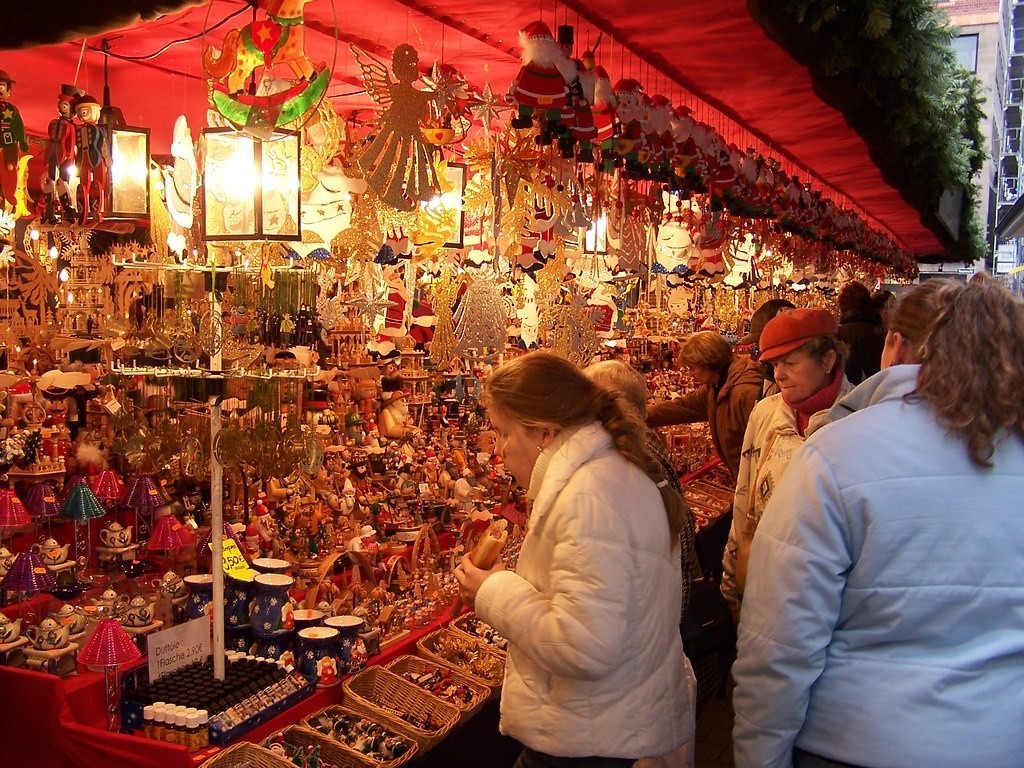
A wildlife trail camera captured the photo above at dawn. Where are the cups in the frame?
[182,560,367,686]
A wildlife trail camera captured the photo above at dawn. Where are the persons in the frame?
[1,69,112,227]
[455,353,701,768]
[9,344,105,480]
[146,351,514,559]
[573,271,1024,768]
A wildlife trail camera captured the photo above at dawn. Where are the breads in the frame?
[469,519,508,570]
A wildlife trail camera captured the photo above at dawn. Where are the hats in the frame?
[758,308,838,361]
[736,299,796,346]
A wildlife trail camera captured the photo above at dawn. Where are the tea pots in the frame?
[0,572,189,651]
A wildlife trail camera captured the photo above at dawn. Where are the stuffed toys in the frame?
[377,262,438,355]
[504,22,919,280]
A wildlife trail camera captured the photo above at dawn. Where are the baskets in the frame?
[416,628,507,700]
[679,462,737,536]
[383,654,492,722]
[198,741,300,768]
[448,611,509,657]
[299,704,419,768]
[258,724,380,768]
[341,665,461,757]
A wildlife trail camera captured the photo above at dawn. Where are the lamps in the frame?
[583,210,608,255]
[194,123,305,242]
[44,38,150,218]
[413,161,468,246]
